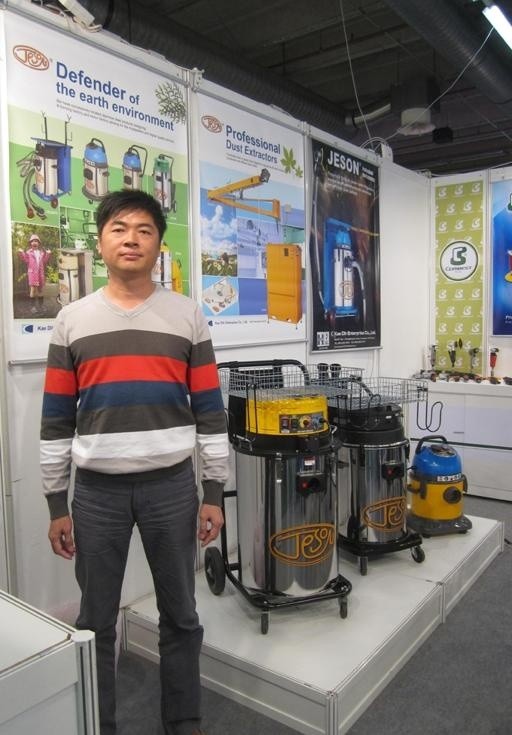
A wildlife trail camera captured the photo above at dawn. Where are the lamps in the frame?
[479,0,512,51]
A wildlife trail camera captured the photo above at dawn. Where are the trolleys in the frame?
[300,363,428,574]
[204,361,350,636]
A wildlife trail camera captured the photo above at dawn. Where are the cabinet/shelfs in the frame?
[404,379,512,501]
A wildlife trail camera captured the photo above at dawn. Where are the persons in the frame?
[38,188,231,735]
[17,235,51,315]
